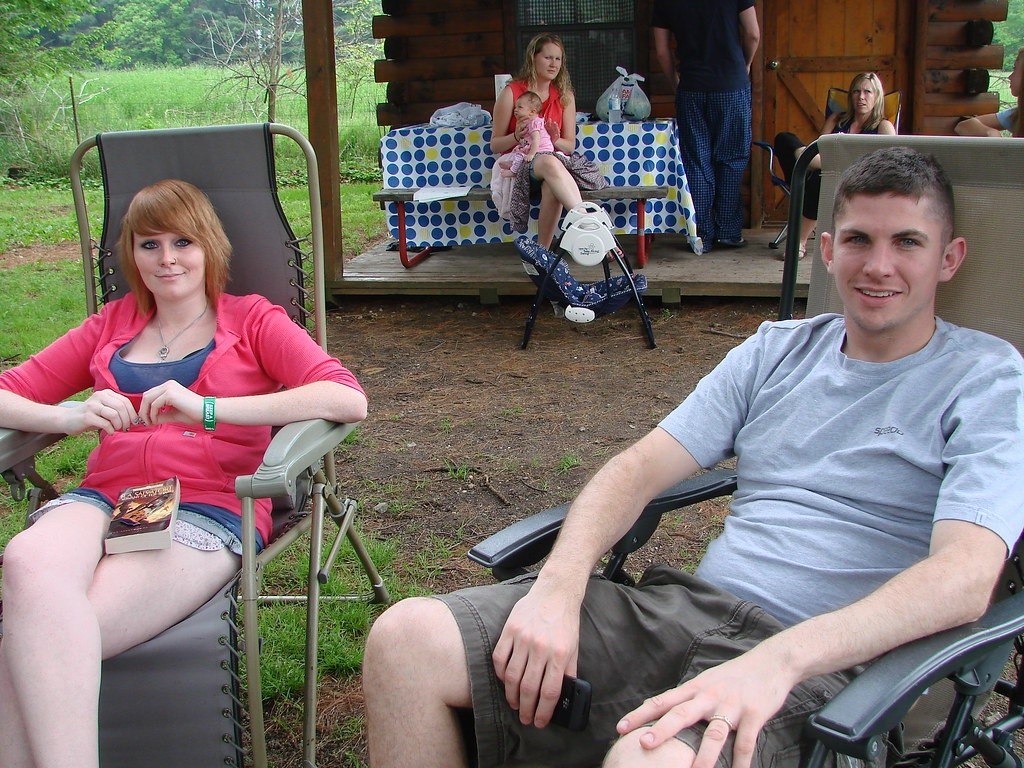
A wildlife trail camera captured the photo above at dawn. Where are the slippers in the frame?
[781,247,807,261]
[718,238,749,247]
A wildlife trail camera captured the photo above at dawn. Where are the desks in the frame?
[380,116,703,258]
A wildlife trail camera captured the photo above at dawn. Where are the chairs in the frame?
[514,201,655,348]
[752,87,903,248]
[468,133,1024,768]
[0,122,387,768]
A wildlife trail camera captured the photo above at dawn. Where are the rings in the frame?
[98,405,105,416]
[708,715,733,730]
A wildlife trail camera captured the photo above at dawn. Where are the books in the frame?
[104,476,181,554]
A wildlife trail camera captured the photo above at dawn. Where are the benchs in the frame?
[373,185,668,267]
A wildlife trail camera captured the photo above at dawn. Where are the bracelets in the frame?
[203,397,216,431]
[513,131,520,143]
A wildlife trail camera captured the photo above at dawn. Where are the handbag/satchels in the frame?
[596,67,651,121]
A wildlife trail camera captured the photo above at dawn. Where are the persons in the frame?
[499,91,554,178]
[774,71,896,260]
[362,147,1023,768]
[490,31,624,262]
[0,180,369,768]
[652,0,761,252]
[955,47,1024,140]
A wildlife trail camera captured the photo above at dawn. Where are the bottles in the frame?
[464,113,490,128]
[609,88,621,123]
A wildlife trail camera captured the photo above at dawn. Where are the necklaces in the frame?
[154,296,208,360]
